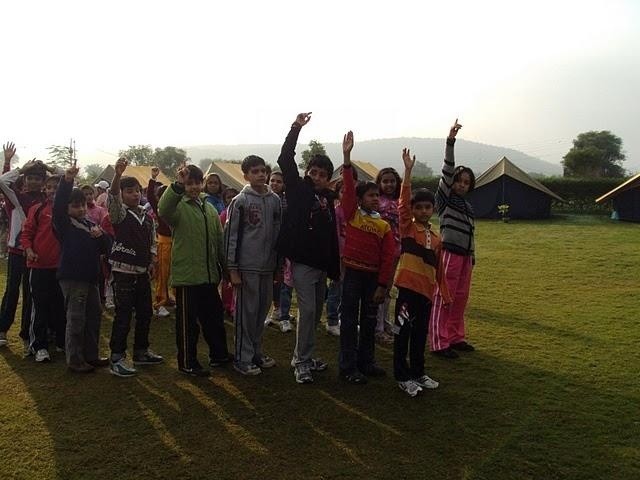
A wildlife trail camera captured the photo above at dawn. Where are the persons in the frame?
[393,146,452,398]
[0,111,413,386]
[428,118,475,360]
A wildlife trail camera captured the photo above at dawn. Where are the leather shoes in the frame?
[431,341,473,358]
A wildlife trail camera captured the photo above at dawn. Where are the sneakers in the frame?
[264,315,295,333]
[365,364,386,377]
[105,295,116,311]
[410,376,440,389]
[325,324,341,337]
[208,353,234,366]
[294,358,328,384]
[68,352,163,377]
[375,319,392,348]
[178,364,209,375]
[23,340,50,362]
[399,380,424,398]
[234,352,275,375]
[153,306,170,317]
[345,372,367,384]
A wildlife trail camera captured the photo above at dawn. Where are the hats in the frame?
[94,181,109,189]
